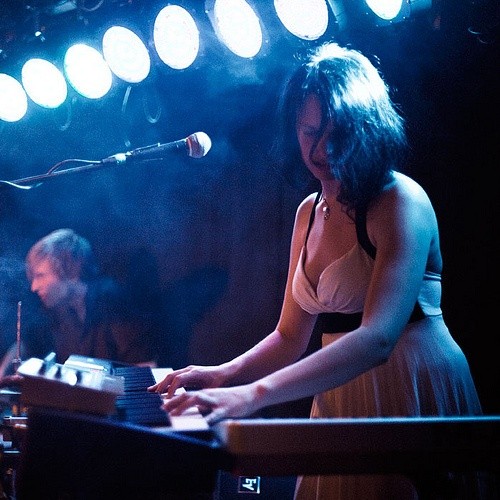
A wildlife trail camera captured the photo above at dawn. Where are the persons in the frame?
[0,228,160,385]
[146,42,485,500]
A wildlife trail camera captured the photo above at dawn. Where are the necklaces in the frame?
[320,191,338,220]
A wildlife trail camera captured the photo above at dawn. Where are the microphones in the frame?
[102,132,211,166]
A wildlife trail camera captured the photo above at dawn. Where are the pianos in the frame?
[10,351,225,458]
